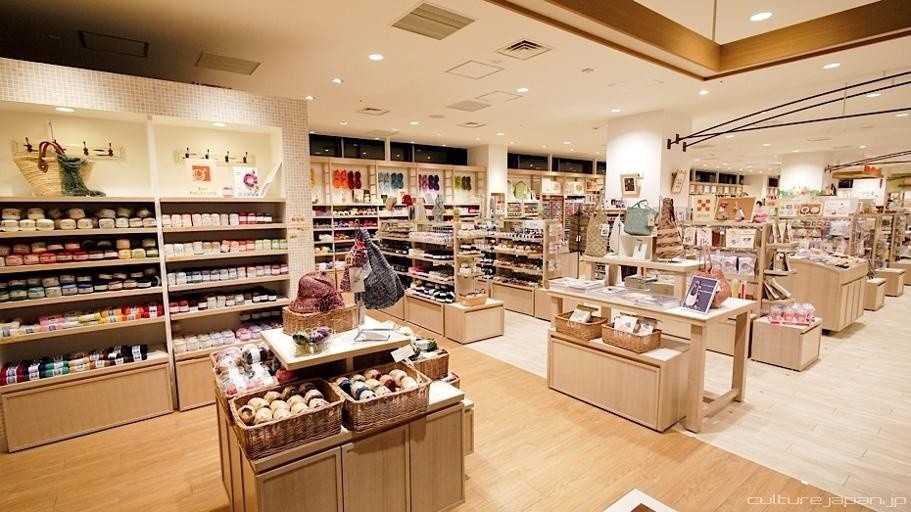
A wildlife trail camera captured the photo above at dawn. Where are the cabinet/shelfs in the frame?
[311,200,547,344]
[549,204,910,435]
[203,318,478,512]
[0,196,175,462]
[157,195,299,413]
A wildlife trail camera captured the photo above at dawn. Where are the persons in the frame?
[901,236,911,256]
[747,200,768,224]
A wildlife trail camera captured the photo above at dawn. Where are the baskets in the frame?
[328,359,433,436]
[281,292,366,336]
[207,341,300,422]
[598,322,663,357]
[409,343,453,383]
[458,289,488,308]
[227,375,347,459]
[553,309,609,343]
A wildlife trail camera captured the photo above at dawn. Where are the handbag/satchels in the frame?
[567,204,590,253]
[583,192,609,258]
[622,198,659,237]
[653,197,687,261]
[12,139,94,199]
[354,228,407,312]
[696,242,733,310]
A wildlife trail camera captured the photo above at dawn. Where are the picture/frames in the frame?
[620,172,640,198]
